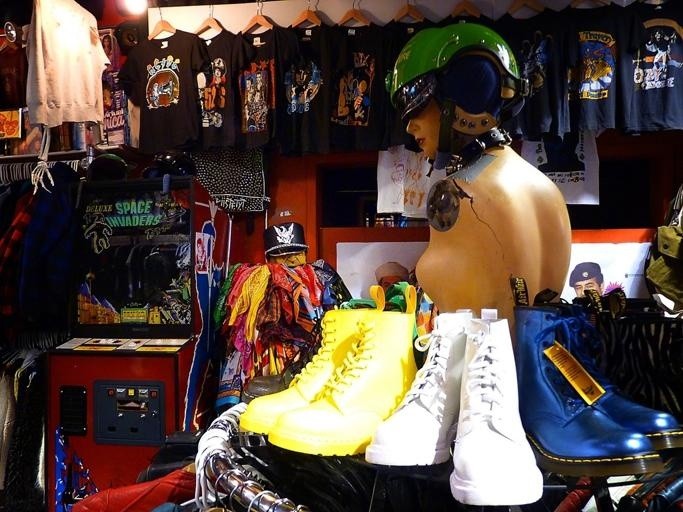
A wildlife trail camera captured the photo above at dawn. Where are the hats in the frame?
[374,261,410,284]
[568,261,601,287]
[263,222,309,258]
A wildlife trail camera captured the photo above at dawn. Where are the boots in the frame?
[532,288,682,452]
[506,276,667,477]
[445,307,545,507]
[362,307,474,468]
[266,284,419,461]
[239,284,385,436]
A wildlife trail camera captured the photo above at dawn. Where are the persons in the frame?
[384,21,573,357]
[567,260,604,300]
[374,259,410,291]
[262,221,309,267]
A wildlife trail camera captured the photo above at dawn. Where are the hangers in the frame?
[331,1,392,42]
[143,0,199,41]
[436,2,497,32]
[381,1,444,44]
[189,2,236,41]
[495,2,547,34]
[286,1,340,41]
[235,1,297,41]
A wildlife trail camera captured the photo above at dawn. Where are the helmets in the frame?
[383,18,521,110]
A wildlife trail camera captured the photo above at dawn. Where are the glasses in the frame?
[392,70,441,129]
